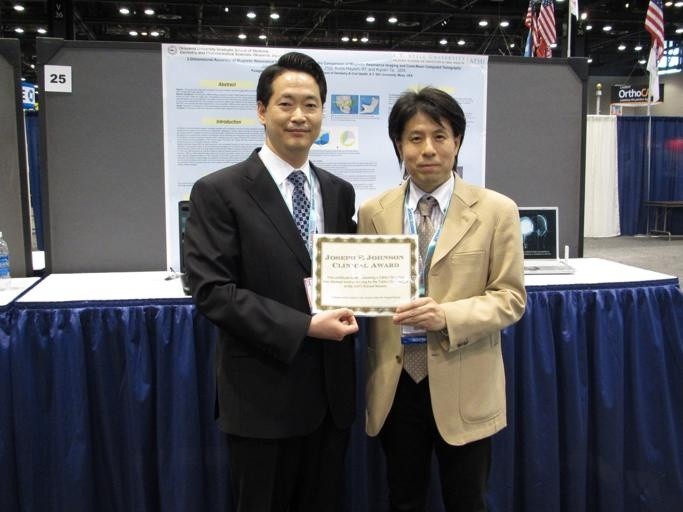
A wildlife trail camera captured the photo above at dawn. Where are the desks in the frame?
[11,257,681,511]
[0,276,49,510]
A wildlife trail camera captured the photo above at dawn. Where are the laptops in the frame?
[518,206,575,274]
[178,200,192,295]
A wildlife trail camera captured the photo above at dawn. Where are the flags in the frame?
[524,2,581,58]
[645,0,667,104]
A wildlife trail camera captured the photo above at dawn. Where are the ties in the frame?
[287,170,310,251]
[403,196,437,384]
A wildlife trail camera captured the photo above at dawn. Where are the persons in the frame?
[188,52,357,511]
[356,89,526,511]
